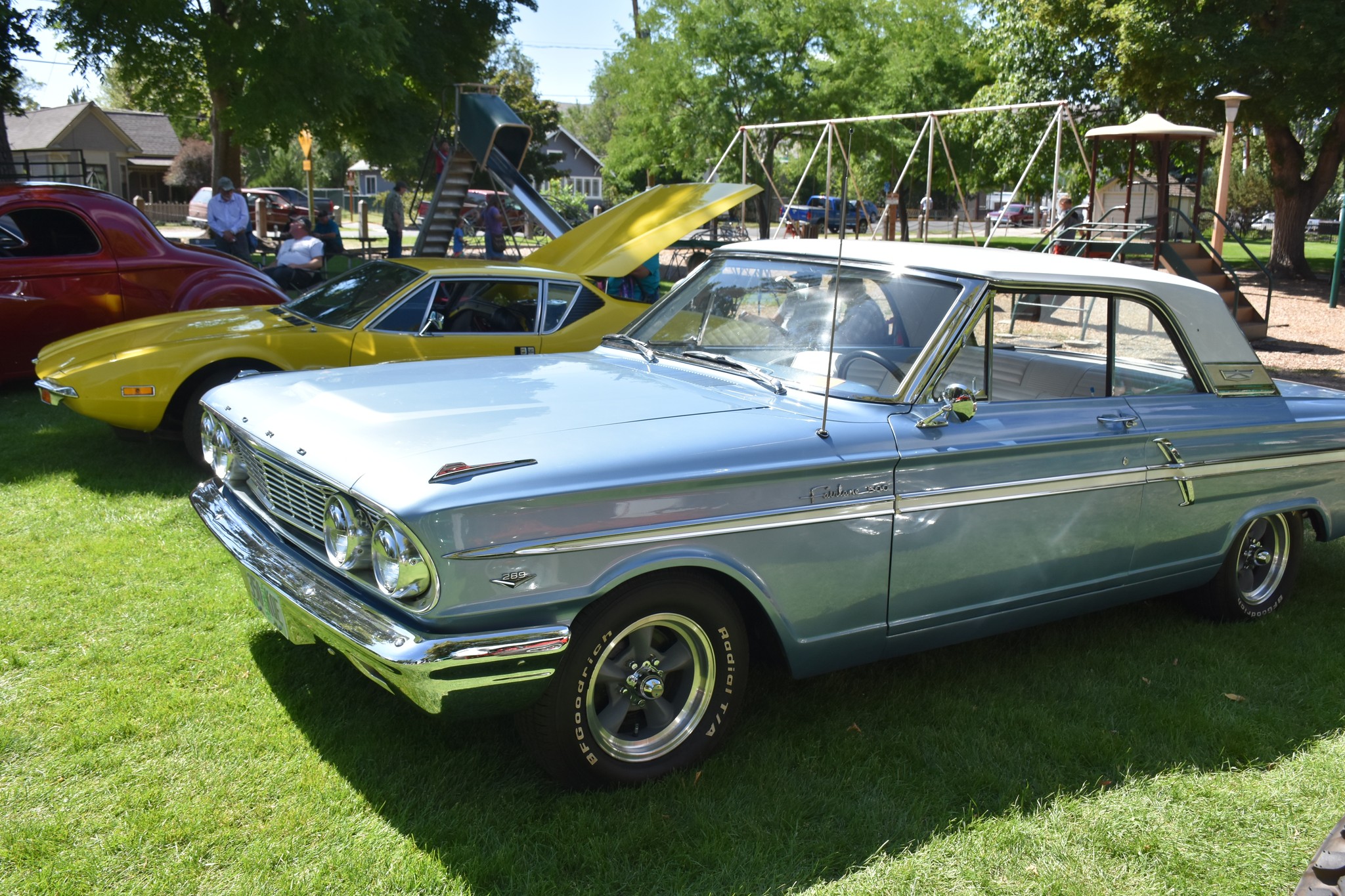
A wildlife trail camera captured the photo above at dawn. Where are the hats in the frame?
[396,181,409,189]
[289,215,315,230]
[218,177,235,191]
[317,207,329,217]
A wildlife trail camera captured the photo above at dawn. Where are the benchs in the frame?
[789,345,1194,401]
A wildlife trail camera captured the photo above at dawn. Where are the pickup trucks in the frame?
[779,195,869,234]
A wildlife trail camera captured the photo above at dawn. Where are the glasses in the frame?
[221,188,234,192]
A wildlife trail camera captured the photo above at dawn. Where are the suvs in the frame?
[186,184,338,234]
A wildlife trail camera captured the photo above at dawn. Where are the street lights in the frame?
[1210,90,1253,267]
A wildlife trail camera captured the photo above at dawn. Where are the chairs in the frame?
[259,240,332,295]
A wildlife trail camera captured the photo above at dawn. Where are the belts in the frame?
[240,230,245,233]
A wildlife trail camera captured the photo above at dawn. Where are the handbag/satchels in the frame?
[491,232,506,253]
[642,285,661,303]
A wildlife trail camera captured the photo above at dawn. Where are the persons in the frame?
[206,176,251,262]
[260,215,324,291]
[1048,206,1058,224]
[920,196,933,224]
[671,252,733,318]
[284,210,311,233]
[311,210,343,265]
[452,219,469,258]
[1042,199,1084,255]
[606,252,660,305]
[484,195,518,260]
[382,182,406,258]
[738,270,890,355]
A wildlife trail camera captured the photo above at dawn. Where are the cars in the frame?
[849,199,880,224]
[187,239,1345,795]
[1,181,292,384]
[32,181,780,471]
[987,204,1043,224]
